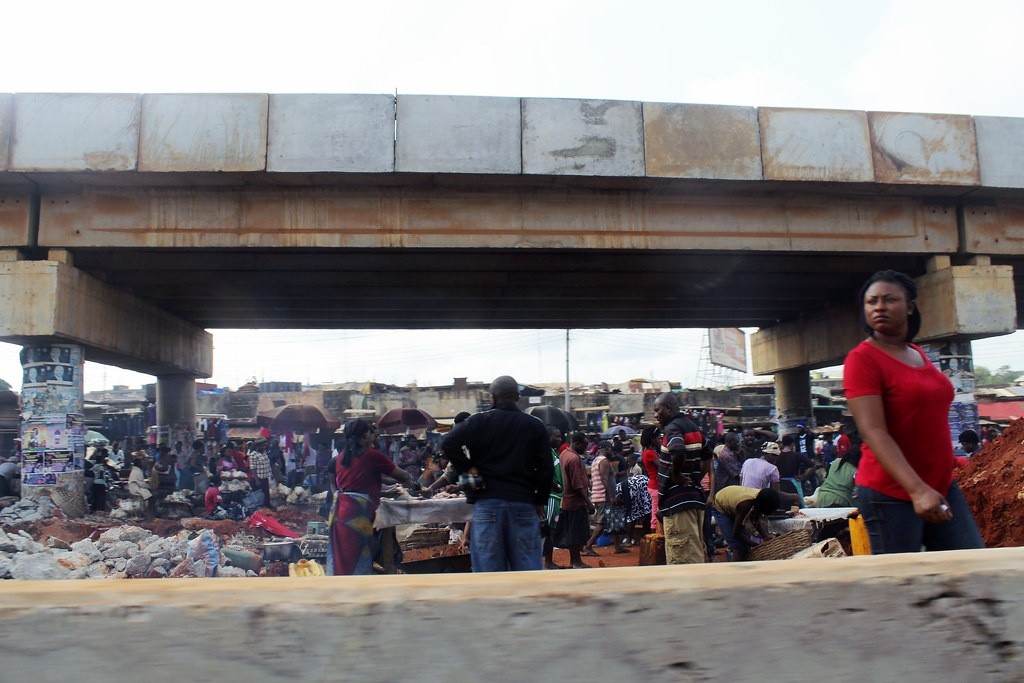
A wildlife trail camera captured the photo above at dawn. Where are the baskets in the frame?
[751,529,811,560]
[51,487,88,519]
[399,528,450,551]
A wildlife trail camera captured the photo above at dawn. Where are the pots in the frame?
[256,541,294,561]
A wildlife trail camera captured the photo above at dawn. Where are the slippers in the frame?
[581,549,601,557]
[614,548,631,554]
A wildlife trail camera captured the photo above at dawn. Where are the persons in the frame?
[327,417,413,575]
[539,425,665,569]
[74,438,342,515]
[958,425,998,459]
[842,271,987,554]
[441,376,556,572]
[23,349,37,363]
[0,439,21,497]
[28,429,39,447]
[49,366,71,381]
[653,393,714,565]
[25,367,46,382]
[702,410,864,563]
[49,347,62,362]
[369,412,485,555]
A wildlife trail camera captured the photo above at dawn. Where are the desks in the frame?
[710,507,860,543]
[372,493,473,575]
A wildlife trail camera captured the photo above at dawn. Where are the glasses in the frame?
[607,445,613,449]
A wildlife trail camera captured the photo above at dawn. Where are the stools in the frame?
[308,522,326,534]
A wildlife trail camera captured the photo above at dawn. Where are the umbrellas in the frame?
[521,405,581,432]
[601,425,636,439]
[374,409,439,445]
[83,430,109,446]
[257,405,342,457]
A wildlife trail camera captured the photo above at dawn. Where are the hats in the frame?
[761,442,781,455]
[796,420,806,428]
[598,440,612,448]
[255,436,267,444]
